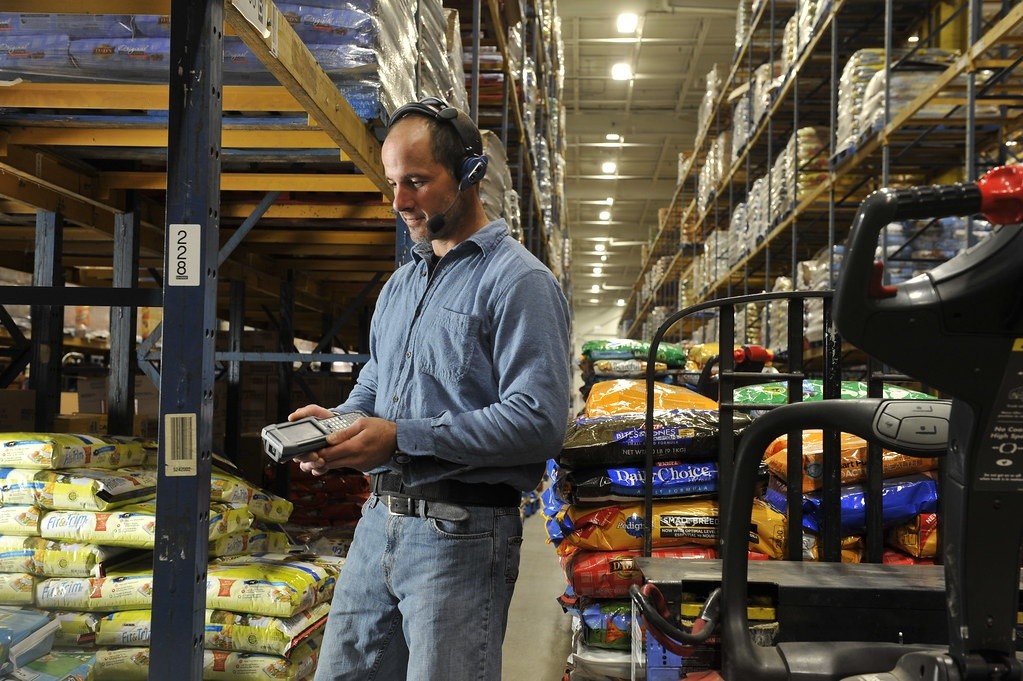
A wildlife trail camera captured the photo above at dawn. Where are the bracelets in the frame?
[333,411,340,417]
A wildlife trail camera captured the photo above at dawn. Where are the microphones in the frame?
[425,185,465,234]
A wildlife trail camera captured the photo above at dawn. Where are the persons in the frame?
[286,97,571,681]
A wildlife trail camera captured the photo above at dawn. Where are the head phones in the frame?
[387,96,488,234]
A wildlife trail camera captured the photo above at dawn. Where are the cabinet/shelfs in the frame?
[0,0,1023,681]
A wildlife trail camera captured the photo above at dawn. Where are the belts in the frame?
[368,472,522,521]
